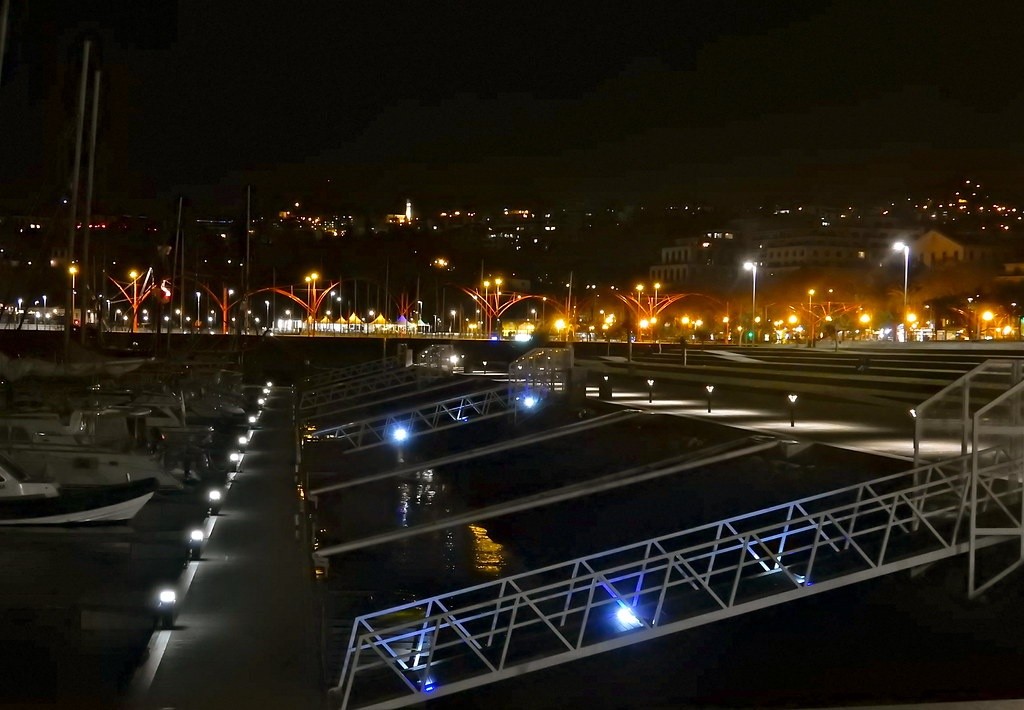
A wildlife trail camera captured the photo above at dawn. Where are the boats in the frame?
[0,372,248,527]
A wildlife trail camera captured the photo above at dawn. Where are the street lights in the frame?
[482,280,492,338]
[646,380,654,403]
[68,265,80,329]
[265,300,270,326]
[494,277,503,330]
[788,391,798,426]
[743,259,757,320]
[635,283,643,327]
[894,240,909,308]
[305,271,320,337]
[807,289,816,323]
[417,300,422,320]
[910,407,917,448]
[705,385,714,414]
[196,291,201,334]
[482,361,487,373]
[129,268,139,333]
[653,282,661,313]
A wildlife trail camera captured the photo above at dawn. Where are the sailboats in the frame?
[0,38,250,433]
